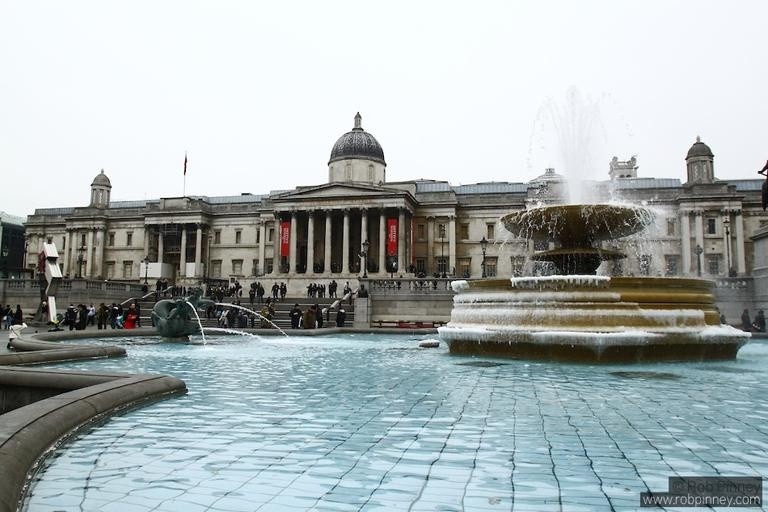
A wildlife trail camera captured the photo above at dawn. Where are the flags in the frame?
[183,157,187,174]
[281,222,289,257]
[387,218,399,257]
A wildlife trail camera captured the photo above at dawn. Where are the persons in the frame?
[42,277,352,330]
[741,308,752,332]
[755,310,765,331]
[0,304,28,349]
[720,314,726,324]
[730,267,736,277]
[665,269,672,277]
[314,261,354,273]
[397,264,469,289]
[358,283,368,297]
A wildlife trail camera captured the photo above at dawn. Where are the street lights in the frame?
[77,244,85,278]
[206,235,212,286]
[362,239,371,278]
[439,228,445,275]
[722,217,732,277]
[2,245,10,270]
[479,236,489,278]
[694,245,702,276]
[144,254,151,285]
